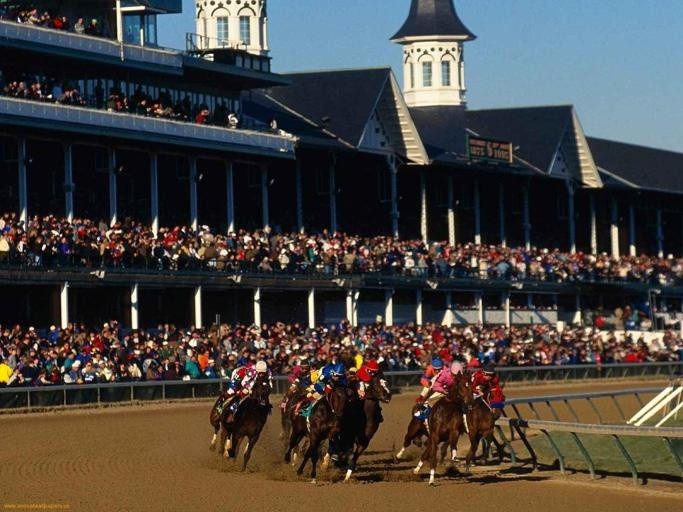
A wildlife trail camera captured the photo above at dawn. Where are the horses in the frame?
[209,370,271,472]
[278,357,392,484]
[392,370,505,487]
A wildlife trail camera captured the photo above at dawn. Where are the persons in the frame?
[262,119,281,136]
[216,360,273,415]
[280,348,392,423]
[2,209,682,286]
[1,0,240,129]
[1,289,683,408]
[414,354,506,420]
